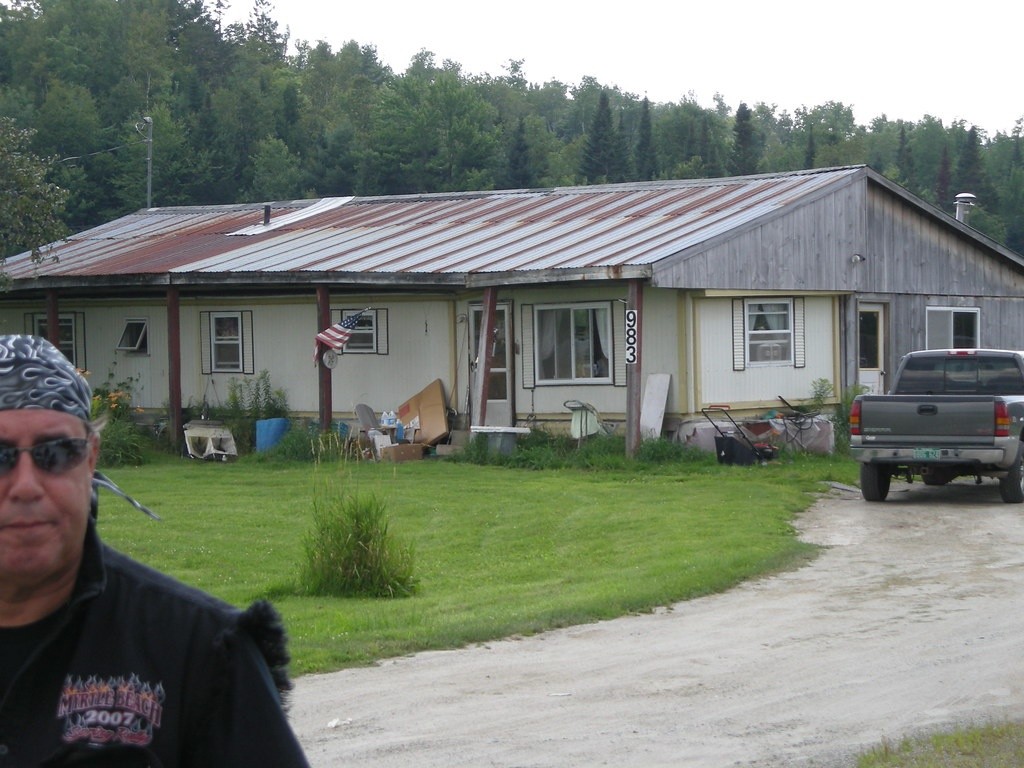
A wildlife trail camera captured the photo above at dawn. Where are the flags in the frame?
[314,309,367,368]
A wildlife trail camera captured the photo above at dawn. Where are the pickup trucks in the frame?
[849,349,1024,503]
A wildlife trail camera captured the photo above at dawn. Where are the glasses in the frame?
[0,437,89,475]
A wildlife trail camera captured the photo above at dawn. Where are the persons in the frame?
[0,330,313,768]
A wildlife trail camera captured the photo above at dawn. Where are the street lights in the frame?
[143,117,152,209]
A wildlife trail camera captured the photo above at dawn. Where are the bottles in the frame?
[381,412,404,444]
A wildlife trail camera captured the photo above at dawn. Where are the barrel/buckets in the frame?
[256,417,290,452]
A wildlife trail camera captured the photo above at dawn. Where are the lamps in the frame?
[851,254,865,264]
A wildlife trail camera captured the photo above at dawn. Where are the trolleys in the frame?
[701,404,766,467]
